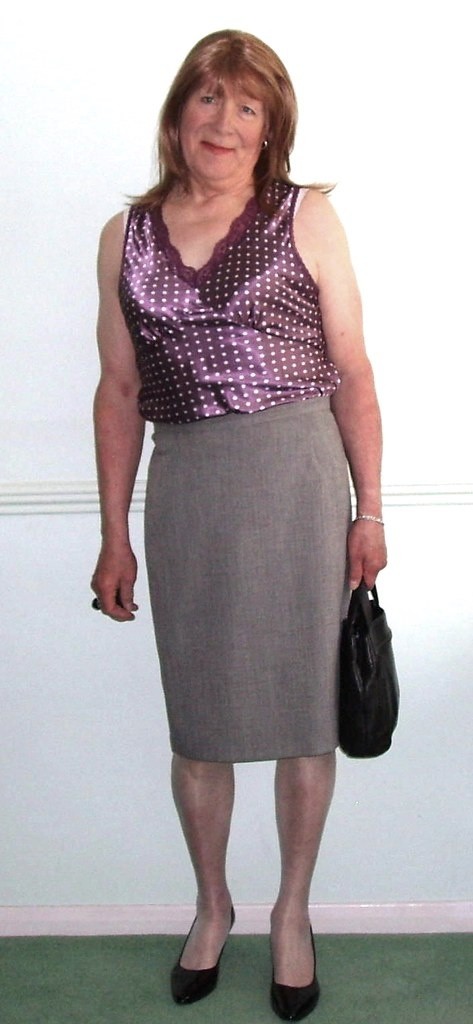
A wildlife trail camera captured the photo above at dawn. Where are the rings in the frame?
[91,599,100,609]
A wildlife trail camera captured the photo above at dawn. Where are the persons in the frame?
[90,30,389,1024]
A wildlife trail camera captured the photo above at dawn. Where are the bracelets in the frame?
[355,514,385,526]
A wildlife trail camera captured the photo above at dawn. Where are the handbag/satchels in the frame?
[338,575,400,758]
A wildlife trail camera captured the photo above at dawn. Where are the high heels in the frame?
[171,903,235,1005]
[271,922,320,1023]
